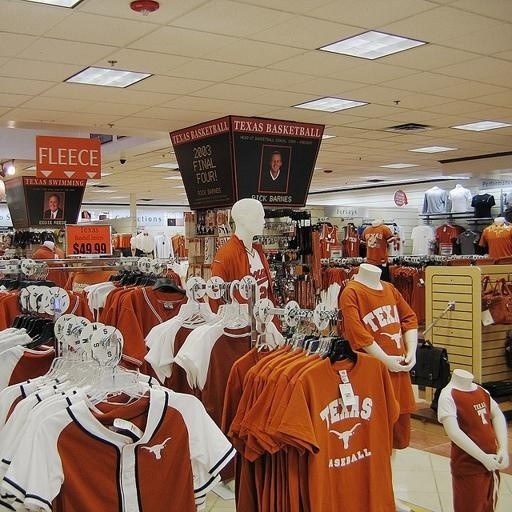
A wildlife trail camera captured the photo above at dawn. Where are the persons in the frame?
[448,183,471,212]
[339,262,420,451]
[44,194,63,218]
[478,216,512,258]
[206,196,284,338]
[359,220,393,264]
[261,149,287,191]
[422,185,448,214]
[437,368,509,511]
[31,240,56,267]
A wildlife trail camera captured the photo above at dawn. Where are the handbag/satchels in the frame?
[481,276,511,327]
[409,338,450,389]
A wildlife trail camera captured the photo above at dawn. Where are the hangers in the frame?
[0,216,487,415]
